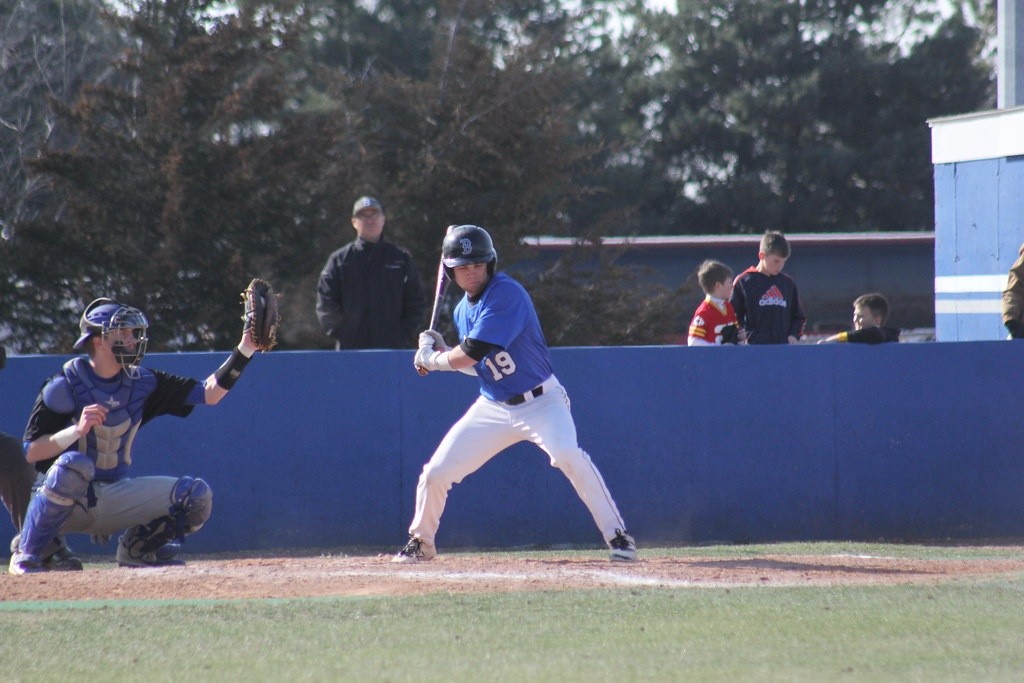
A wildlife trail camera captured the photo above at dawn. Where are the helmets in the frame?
[72,297,149,350]
[442,224,498,281]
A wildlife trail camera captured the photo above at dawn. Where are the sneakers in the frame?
[391,534,436,563]
[608,528,637,561]
[9,533,49,575]
[116,535,182,565]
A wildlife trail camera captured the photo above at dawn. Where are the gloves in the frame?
[414,330,447,376]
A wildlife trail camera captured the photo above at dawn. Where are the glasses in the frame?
[357,213,381,220]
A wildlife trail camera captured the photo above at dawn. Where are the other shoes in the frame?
[42,547,83,571]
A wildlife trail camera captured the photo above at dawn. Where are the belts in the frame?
[505,386,543,405]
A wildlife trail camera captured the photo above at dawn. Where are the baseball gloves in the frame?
[242,278,281,353]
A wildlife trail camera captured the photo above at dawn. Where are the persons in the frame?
[390,225,637,565]
[1000,243,1024,340]
[0,278,278,576]
[687,230,901,346]
[315,196,427,350]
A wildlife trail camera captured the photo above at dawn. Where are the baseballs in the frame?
[260,296,266,310]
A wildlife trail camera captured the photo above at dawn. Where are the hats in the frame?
[352,195,384,216]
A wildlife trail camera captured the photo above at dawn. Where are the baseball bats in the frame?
[416,224,460,376]
